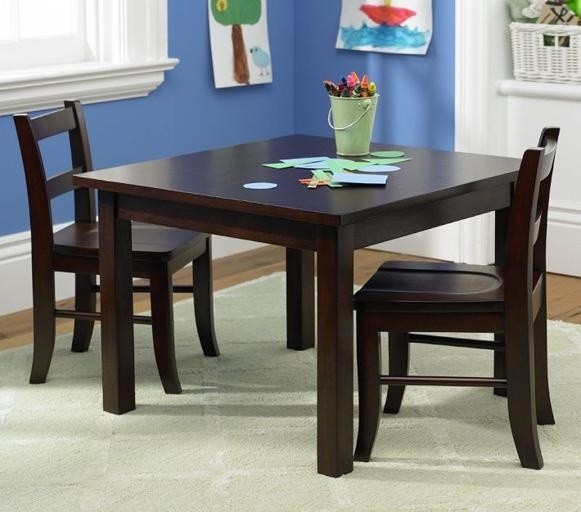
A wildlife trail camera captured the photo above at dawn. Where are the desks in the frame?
[12,100,219,395]
[70,134,522,479]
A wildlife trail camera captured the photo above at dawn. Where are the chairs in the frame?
[351,127,562,470]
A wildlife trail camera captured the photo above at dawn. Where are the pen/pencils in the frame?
[323,72,376,97]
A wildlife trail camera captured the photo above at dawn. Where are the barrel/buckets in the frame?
[327,91,381,157]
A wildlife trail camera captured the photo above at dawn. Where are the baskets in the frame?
[509,22,581,86]
